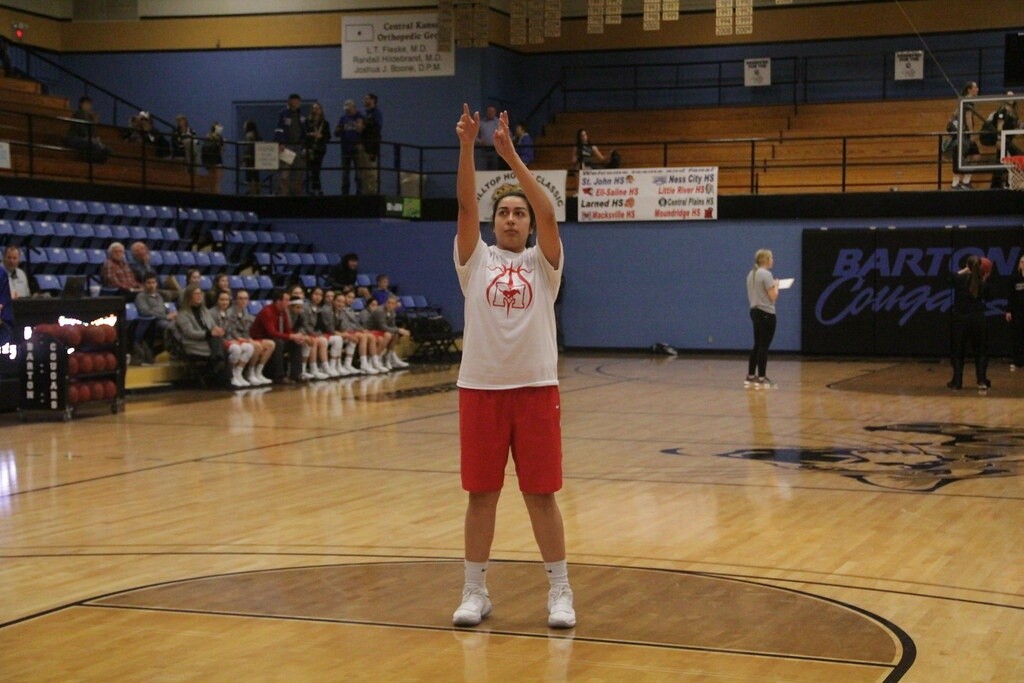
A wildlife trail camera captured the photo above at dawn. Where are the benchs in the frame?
[0,67,212,193]
[526,94,1023,197]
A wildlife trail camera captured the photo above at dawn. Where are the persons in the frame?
[335,88,383,199]
[990,90,1018,188]
[572,128,612,170]
[276,93,331,197]
[746,249,779,385]
[172,115,199,174]
[449,102,576,631]
[950,79,980,192]
[946,256,999,390]
[104,241,413,388]
[202,121,226,194]
[1005,254,1024,372]
[66,95,113,165]
[238,119,263,196]
[0,246,41,309]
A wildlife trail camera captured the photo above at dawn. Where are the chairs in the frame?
[0,193,462,390]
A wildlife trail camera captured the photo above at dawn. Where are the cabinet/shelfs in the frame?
[10,296,127,423]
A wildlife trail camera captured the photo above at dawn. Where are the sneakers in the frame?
[547,589,576,627]
[453,583,492,625]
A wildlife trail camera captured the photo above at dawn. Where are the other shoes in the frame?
[947,373,962,389]
[298,359,409,379]
[977,376,991,387]
[247,371,272,387]
[754,377,776,386]
[230,377,251,387]
[744,374,757,384]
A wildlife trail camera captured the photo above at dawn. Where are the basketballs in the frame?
[980,257,993,272]
[68,381,117,402]
[32,324,117,346]
[67,352,117,374]
